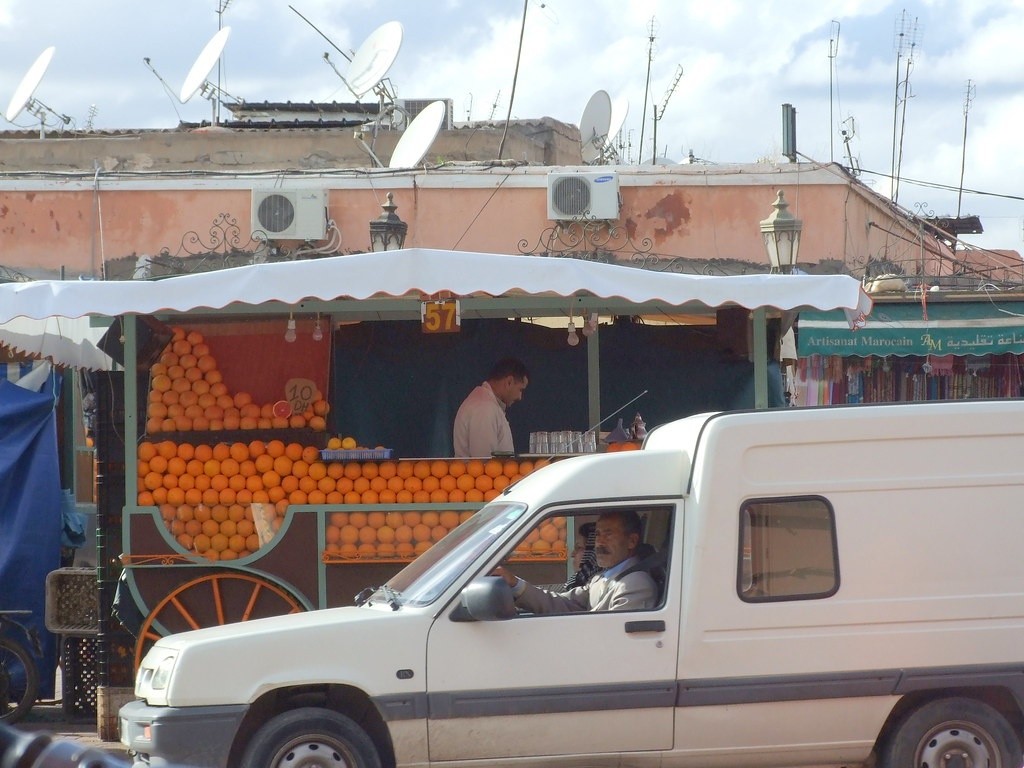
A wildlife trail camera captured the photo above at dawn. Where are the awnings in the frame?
[0,244,876,379]
[795,300,1023,361]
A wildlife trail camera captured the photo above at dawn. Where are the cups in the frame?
[529,429,598,454]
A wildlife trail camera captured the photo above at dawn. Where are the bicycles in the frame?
[0,608,47,727]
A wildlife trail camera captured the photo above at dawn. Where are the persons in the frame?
[452,355,532,459]
[490,509,659,613]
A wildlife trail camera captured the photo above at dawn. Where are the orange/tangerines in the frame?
[136,328,641,563]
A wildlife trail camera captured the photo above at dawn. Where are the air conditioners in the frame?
[545,172,622,222]
[250,186,331,240]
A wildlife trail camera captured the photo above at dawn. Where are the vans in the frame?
[119,394,1024,768]
